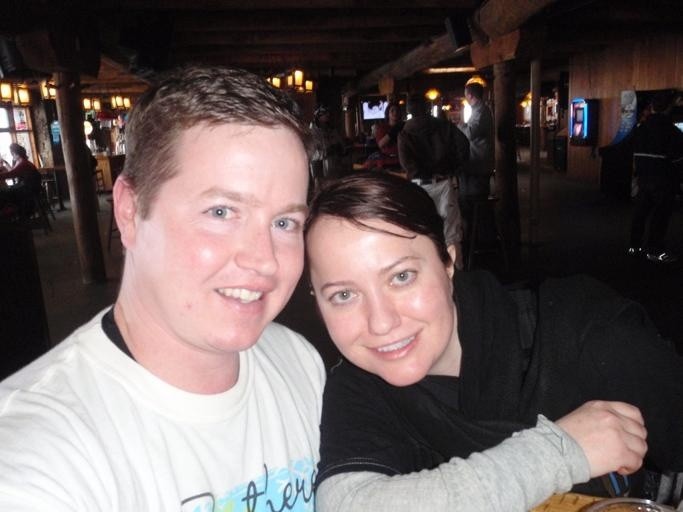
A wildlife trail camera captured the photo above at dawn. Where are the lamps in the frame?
[83,95,131,112]
[0,80,55,107]
[265,67,313,94]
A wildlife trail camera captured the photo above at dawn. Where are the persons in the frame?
[300,169,681,512]
[0,60,338,512]
[625,92,682,264]
[305,82,494,272]
[0,143,42,228]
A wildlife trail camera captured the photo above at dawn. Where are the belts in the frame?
[418,175,448,185]
